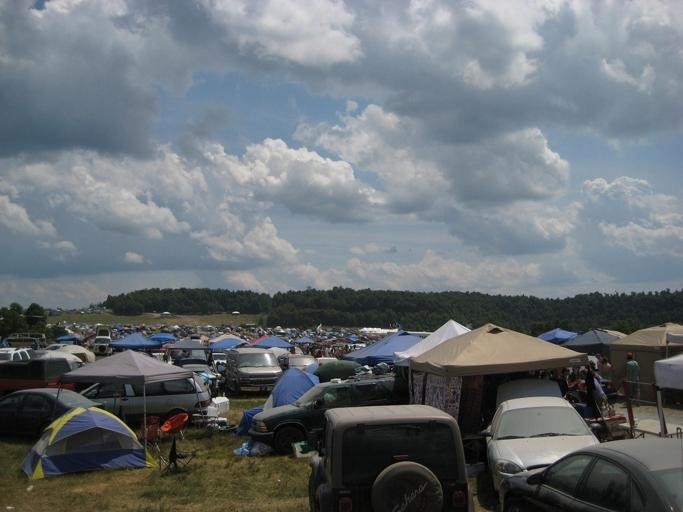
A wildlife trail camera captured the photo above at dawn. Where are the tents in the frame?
[20,404,160,482]
[233,360,364,437]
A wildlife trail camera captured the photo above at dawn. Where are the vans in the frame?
[78,371,212,428]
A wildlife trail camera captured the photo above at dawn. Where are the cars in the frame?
[475,378,600,511]
[0,389,103,436]
[503,437,683,512]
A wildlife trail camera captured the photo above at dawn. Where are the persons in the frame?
[563,352,641,409]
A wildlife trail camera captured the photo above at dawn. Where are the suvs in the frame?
[307,403,475,511]
[248,371,396,455]
